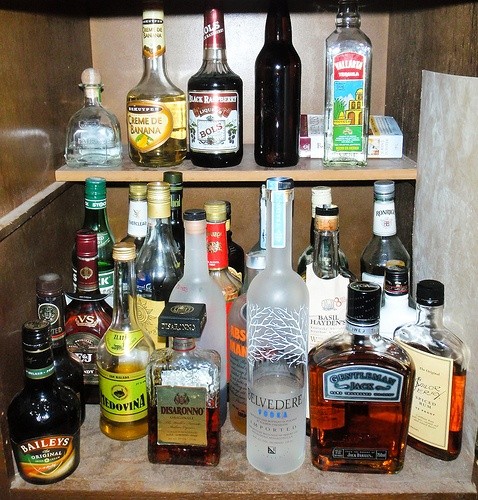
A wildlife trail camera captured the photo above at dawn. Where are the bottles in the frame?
[358,179,408,289]
[253,0,302,166]
[394,278,468,461]
[146,302,221,469]
[299,202,358,436]
[381,259,418,342]
[122,185,146,251]
[187,0,242,168]
[229,177,309,474]
[6,319,80,486]
[127,0,189,167]
[304,282,417,475]
[222,201,244,281]
[95,241,155,441]
[64,66,122,166]
[296,187,349,283]
[35,273,84,428]
[163,171,184,252]
[168,209,228,427]
[321,0,374,167]
[138,181,184,298]
[70,176,115,309]
[66,230,112,403]
[202,198,243,403]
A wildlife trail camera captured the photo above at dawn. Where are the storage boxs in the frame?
[301,115,404,159]
